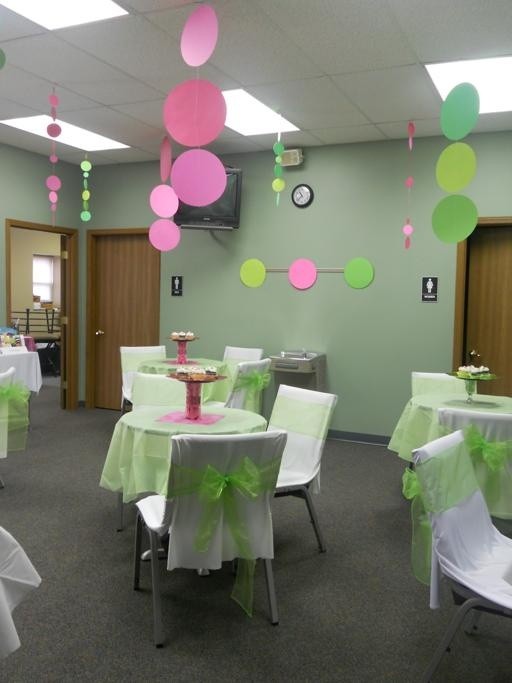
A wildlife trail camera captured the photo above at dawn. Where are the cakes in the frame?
[457,348,490,374]
[173,366,218,382]
[168,331,195,340]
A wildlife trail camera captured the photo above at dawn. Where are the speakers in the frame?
[275,147,306,168]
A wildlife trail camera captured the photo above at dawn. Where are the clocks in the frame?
[291,183,314,209]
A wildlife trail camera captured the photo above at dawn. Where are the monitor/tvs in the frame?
[171,167,245,230]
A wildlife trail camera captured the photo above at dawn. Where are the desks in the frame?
[19,306,62,342]
